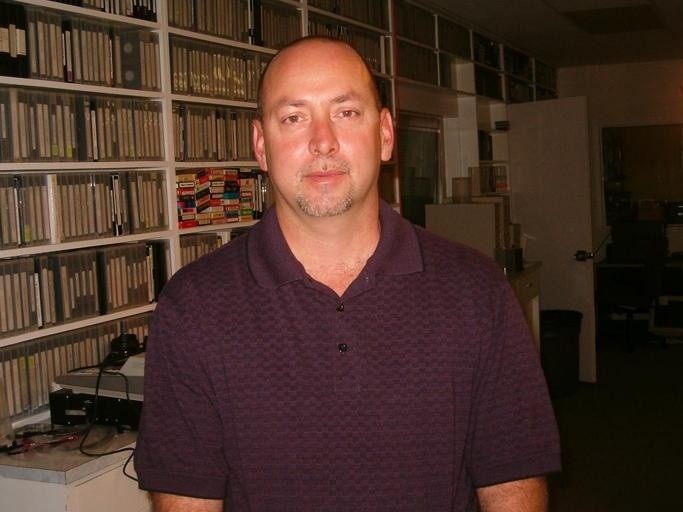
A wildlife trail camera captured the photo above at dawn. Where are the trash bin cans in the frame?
[540,309,583,397]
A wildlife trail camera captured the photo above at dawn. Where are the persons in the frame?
[138,35,563,512]
